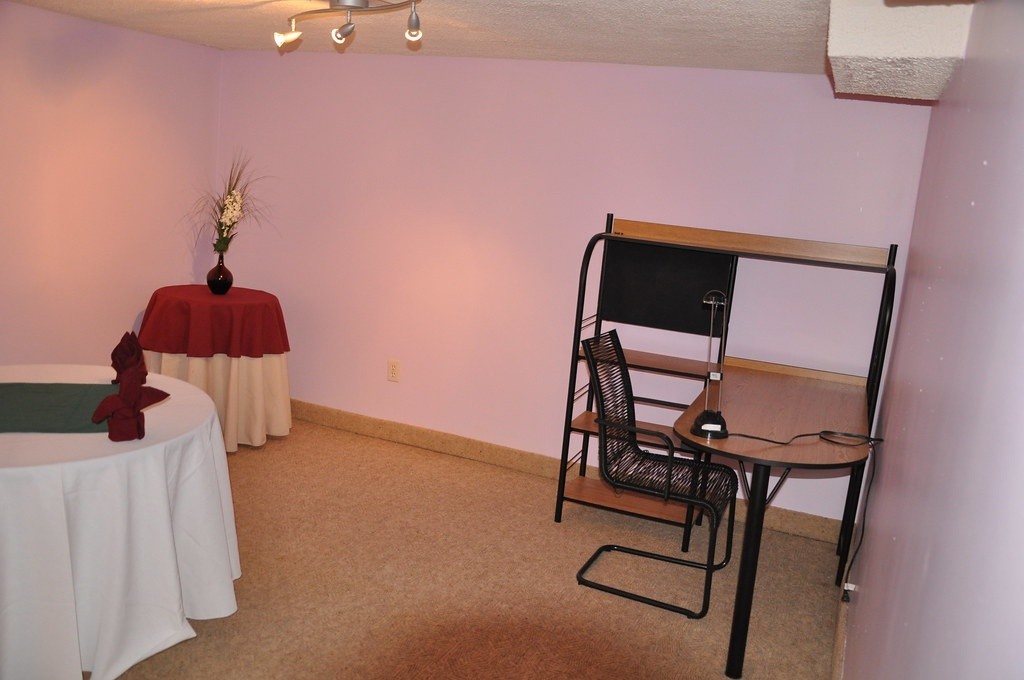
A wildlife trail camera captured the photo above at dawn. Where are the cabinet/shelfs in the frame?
[555,210,899,679]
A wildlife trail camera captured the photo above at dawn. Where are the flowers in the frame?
[177,144,282,254]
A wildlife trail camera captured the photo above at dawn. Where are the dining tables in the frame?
[135,284,292,452]
[1,364,242,680]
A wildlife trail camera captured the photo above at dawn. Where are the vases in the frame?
[207,252,233,295]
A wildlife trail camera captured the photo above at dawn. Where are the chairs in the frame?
[575,328,738,621]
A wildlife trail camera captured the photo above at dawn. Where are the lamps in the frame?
[273,0,422,49]
